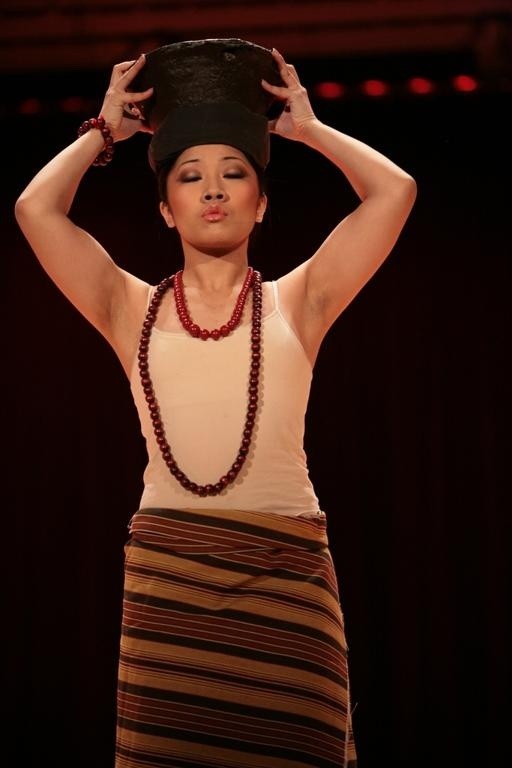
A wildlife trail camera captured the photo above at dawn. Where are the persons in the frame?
[14,47,420,767]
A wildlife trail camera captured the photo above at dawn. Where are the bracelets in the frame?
[77,116,115,168]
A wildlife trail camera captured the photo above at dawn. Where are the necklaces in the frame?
[170,266,251,338]
[137,258,262,499]
